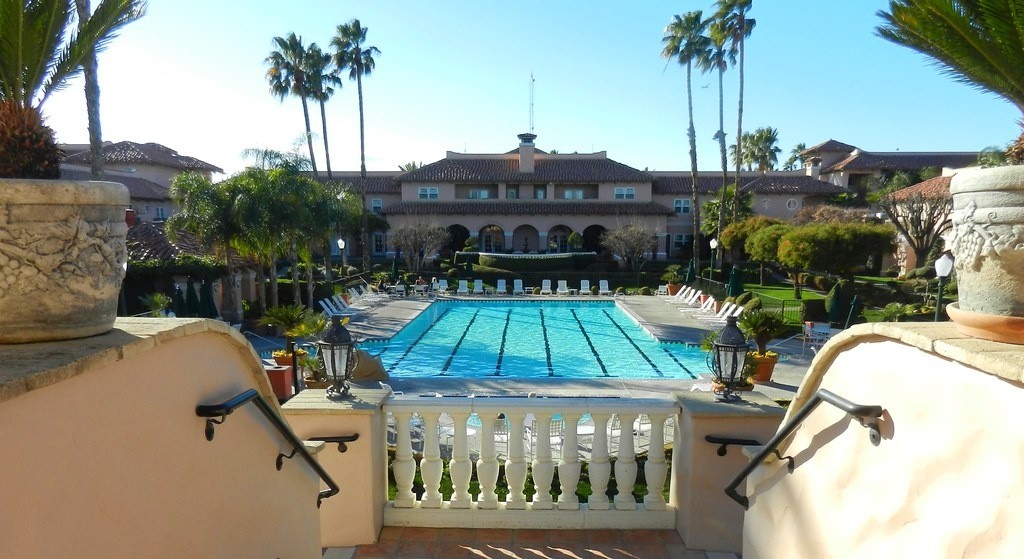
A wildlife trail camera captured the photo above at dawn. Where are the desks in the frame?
[568,288,578,297]
[614,292,626,301]
[802,325,840,356]
[563,425,595,451]
[524,287,534,294]
[410,284,429,287]
[387,286,406,287]
[445,428,477,452]
[485,287,496,296]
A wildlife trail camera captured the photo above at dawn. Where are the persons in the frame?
[431,277,440,294]
[378,278,390,294]
[397,276,410,298]
[415,276,427,298]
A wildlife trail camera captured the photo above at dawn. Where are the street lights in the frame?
[934,253,953,322]
[338,238,346,293]
[707,238,718,294]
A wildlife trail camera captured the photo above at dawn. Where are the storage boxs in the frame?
[805,321,815,328]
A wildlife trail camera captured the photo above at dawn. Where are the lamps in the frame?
[705,316,753,403]
[316,314,360,401]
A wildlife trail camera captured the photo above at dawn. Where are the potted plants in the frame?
[698,321,755,392]
[665,264,684,296]
[297,315,351,388]
[870,0,1024,344]
[0,0,149,346]
[256,304,314,378]
[712,284,726,314]
[700,282,710,305]
[737,309,788,385]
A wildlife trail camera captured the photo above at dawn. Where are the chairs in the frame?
[427,283,440,298]
[654,285,745,326]
[232,323,242,331]
[317,284,392,319]
[378,381,405,397]
[691,382,711,392]
[439,280,450,296]
[457,280,470,296]
[396,284,407,298]
[393,413,675,461]
[513,279,524,296]
[497,279,507,295]
[224,322,231,326]
[540,280,552,296]
[579,279,593,296]
[557,280,569,297]
[598,280,611,296]
[415,285,425,297]
[473,280,484,295]
